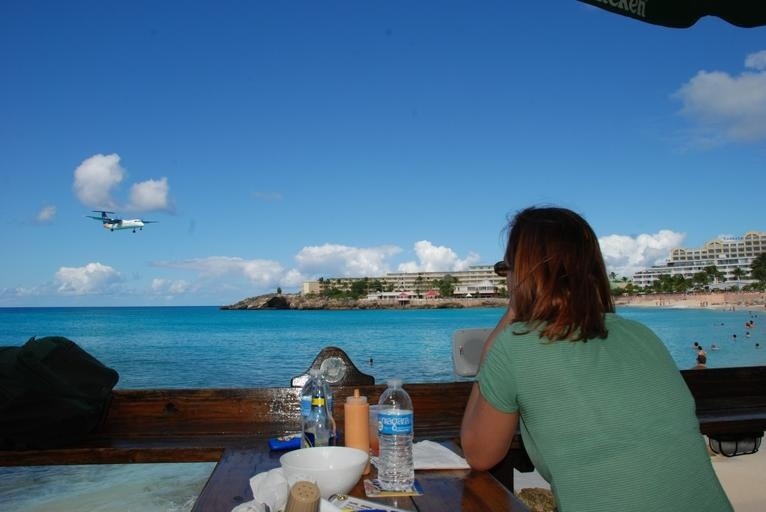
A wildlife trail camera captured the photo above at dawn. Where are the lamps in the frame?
[705,428,765,458]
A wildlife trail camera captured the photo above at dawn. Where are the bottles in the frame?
[302,386,333,450]
[376,380,416,492]
[345,389,371,477]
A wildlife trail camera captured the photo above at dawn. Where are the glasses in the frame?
[492,260,515,278]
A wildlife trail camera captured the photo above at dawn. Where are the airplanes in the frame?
[84,210,158,233]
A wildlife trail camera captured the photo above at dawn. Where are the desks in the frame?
[190,442,533,512]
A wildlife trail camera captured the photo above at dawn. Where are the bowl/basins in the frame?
[279,445,372,504]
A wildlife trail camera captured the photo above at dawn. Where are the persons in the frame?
[691,315,759,368]
[461,207,734,512]
